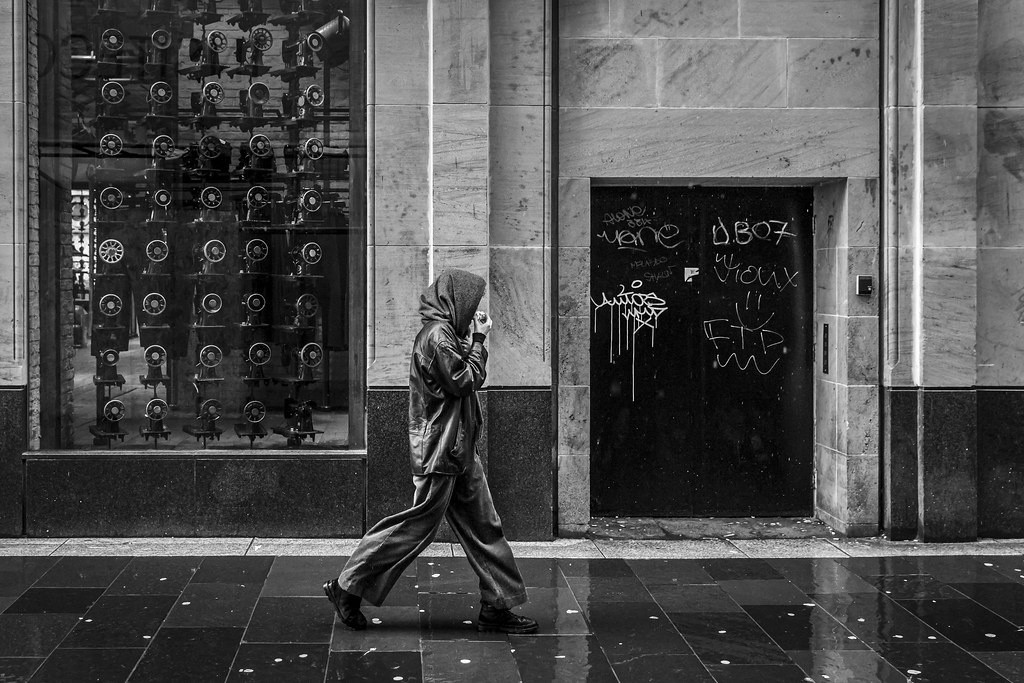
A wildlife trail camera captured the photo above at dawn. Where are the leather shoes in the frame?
[322,578,368,631]
[477,604,539,634]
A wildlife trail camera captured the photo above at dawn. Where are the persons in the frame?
[323,268,539,634]
